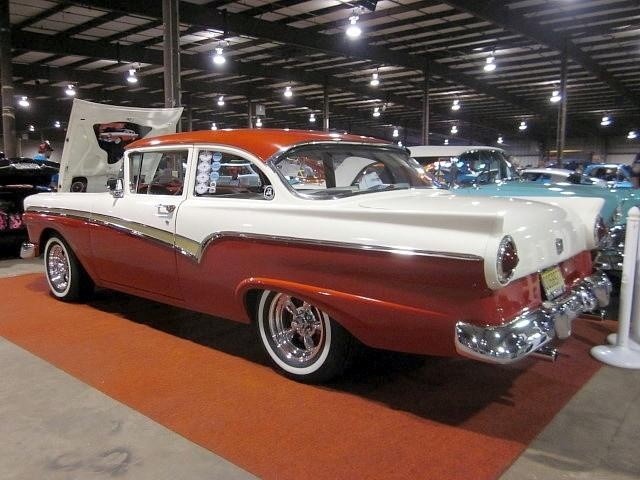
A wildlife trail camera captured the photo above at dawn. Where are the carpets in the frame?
[1,265,635,478]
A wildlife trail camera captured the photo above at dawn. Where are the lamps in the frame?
[518,116,528,131]
[347,8,362,38]
[18,96,30,108]
[450,120,458,135]
[126,66,138,84]
[392,126,400,137]
[373,102,382,119]
[626,124,637,140]
[255,118,263,129]
[211,122,218,131]
[216,95,225,106]
[497,135,503,146]
[308,109,317,123]
[65,83,77,98]
[483,45,497,74]
[284,83,293,98]
[443,137,450,145]
[54,119,61,129]
[396,139,404,147]
[550,82,562,104]
[28,124,35,132]
[600,109,611,126]
[370,68,381,88]
[452,98,461,112]
[213,43,225,66]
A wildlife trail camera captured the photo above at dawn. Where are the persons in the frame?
[32,143,54,162]
